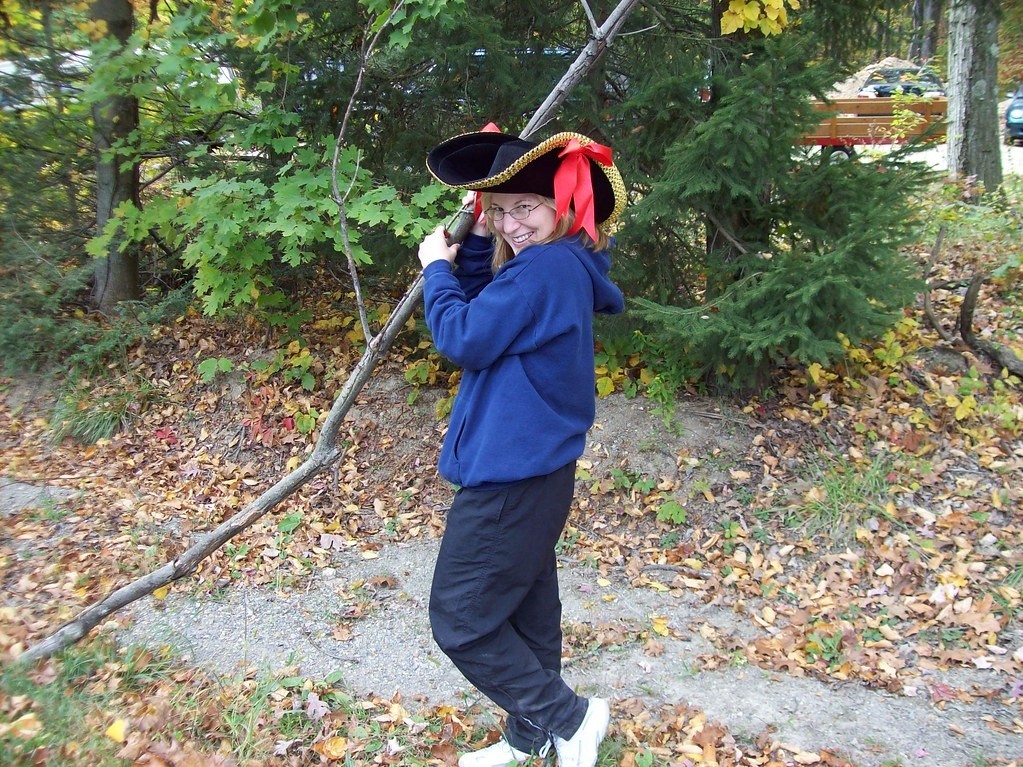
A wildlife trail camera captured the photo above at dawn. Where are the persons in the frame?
[416,130,628,767]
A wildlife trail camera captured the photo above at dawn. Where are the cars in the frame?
[0,37,714,184]
[855,67,949,118]
[1003,83,1023,146]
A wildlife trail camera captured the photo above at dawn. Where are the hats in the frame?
[426,131,627,229]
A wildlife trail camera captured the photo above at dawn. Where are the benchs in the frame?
[789,96,948,163]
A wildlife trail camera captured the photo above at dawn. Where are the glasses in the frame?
[483,202,544,221]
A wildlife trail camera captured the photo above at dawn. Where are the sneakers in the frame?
[458,738,528,767]
[538,698,609,766]
